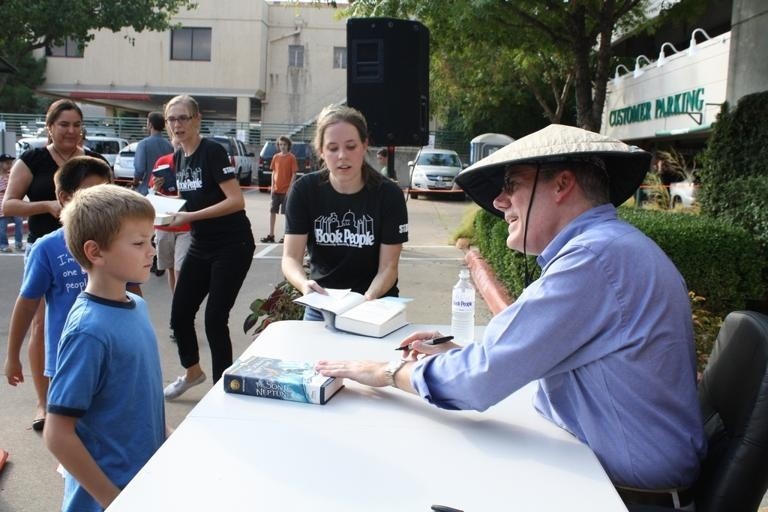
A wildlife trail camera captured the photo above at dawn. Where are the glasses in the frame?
[501,166,558,194]
[165,114,196,123]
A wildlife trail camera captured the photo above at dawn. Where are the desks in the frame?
[105,319,630,512]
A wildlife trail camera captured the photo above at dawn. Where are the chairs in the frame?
[697,309,768,512]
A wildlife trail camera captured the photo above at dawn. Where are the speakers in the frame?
[347,17,430,148]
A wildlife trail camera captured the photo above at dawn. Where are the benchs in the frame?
[636,183,704,208]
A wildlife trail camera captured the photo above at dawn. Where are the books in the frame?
[152,163,178,193]
[223,354,346,406]
[141,192,189,227]
[290,286,410,339]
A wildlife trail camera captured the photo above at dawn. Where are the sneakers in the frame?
[0,242,26,252]
[260,234,275,242]
[162,371,206,399]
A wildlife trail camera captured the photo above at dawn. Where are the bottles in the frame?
[451,269,477,345]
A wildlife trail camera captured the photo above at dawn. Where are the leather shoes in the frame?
[32,418,44,431]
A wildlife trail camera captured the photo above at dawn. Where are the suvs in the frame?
[258,140,318,193]
[203,134,256,185]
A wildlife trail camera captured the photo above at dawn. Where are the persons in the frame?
[145,148,194,341]
[2,155,146,425]
[130,112,175,197]
[39,181,168,512]
[1,97,111,432]
[376,148,400,187]
[161,92,258,401]
[279,99,410,322]
[259,135,300,244]
[103,144,116,154]
[315,121,717,511]
[0,153,27,253]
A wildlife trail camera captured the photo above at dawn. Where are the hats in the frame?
[453,124,654,221]
[0,154,16,162]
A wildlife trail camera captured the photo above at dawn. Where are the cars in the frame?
[665,166,701,208]
[114,143,143,181]
[18,132,126,168]
[410,147,465,201]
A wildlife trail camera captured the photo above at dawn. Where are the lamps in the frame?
[614,28,711,86]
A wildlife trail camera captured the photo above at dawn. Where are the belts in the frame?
[618,486,693,510]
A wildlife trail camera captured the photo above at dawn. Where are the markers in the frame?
[395,335,455,351]
